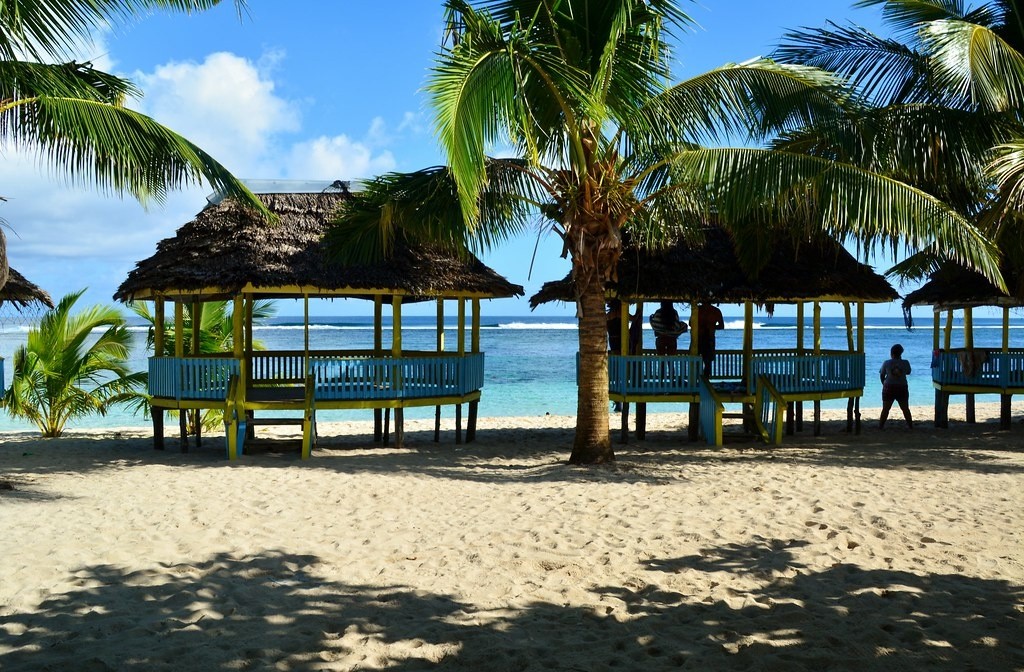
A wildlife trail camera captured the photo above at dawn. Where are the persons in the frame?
[874,344,915,430]
[649,301,688,387]
[689,302,726,380]
[607,300,633,412]
